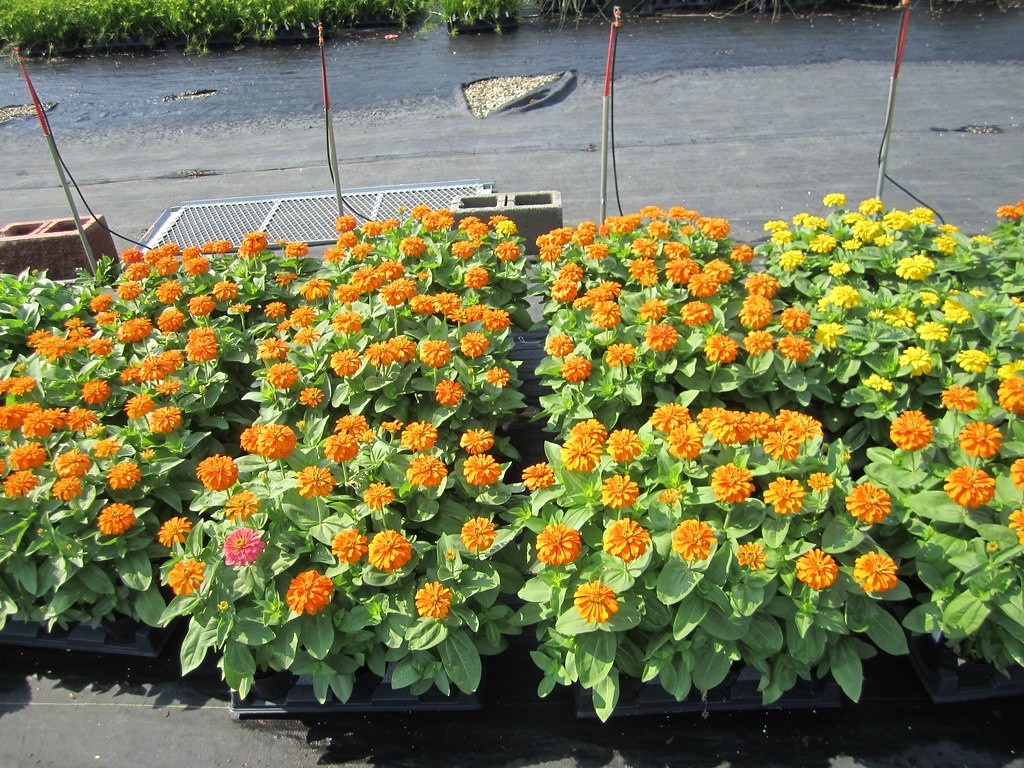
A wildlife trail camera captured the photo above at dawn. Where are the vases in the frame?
[570,668,878,718]
[902,636,1024,712]
[0,622,167,658]
[227,660,480,718]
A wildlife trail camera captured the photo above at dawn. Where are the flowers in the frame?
[0,193,1024,722]
